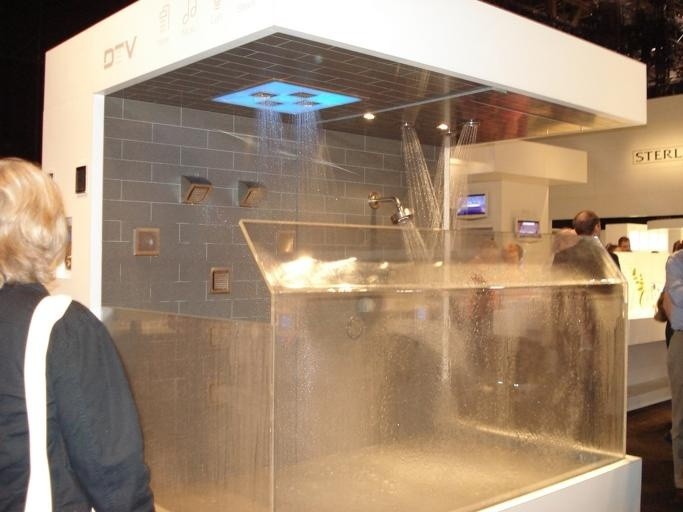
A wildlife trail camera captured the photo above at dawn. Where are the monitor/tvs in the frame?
[457,193,485,215]
[516,218,542,242]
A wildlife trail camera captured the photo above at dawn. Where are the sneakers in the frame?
[673,488,683,504]
[663,430,673,443]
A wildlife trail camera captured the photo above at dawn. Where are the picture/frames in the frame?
[133,227,161,256]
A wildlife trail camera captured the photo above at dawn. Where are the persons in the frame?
[665,248,682,502]
[0,155,154,510]
[653,239,683,443]
[451,226,581,400]
[617,234,631,250]
[550,209,623,444]
